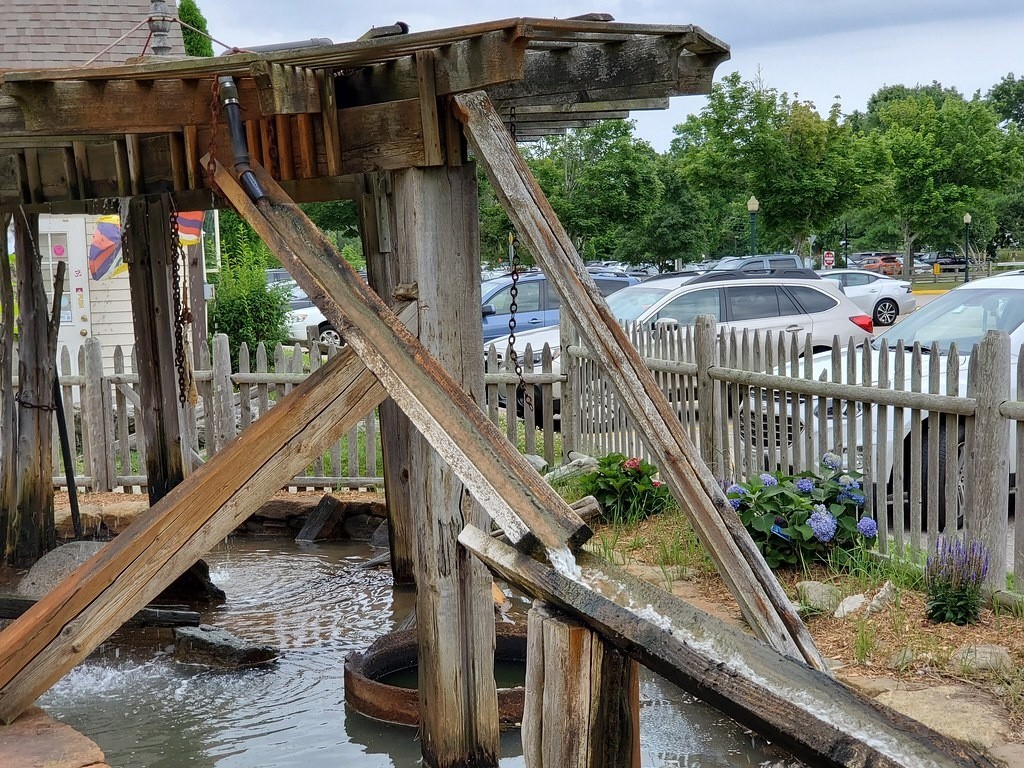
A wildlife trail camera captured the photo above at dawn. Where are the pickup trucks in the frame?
[917,250,966,272]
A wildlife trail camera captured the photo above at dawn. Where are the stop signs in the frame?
[823,251,835,267]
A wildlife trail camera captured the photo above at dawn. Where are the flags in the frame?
[174,210,204,246]
[90,216,128,281]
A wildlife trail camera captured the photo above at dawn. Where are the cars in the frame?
[740,269,1023,532]
[814,270,916,326]
[587,260,659,276]
[264,268,368,310]
[849,252,889,264]
[709,255,804,272]
[896,258,933,274]
[279,306,345,355]
[857,256,903,275]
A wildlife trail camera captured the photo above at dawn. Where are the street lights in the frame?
[748,196,759,256]
[964,213,972,283]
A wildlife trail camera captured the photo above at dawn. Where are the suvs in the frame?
[481,270,655,343]
[484,268,873,434]
